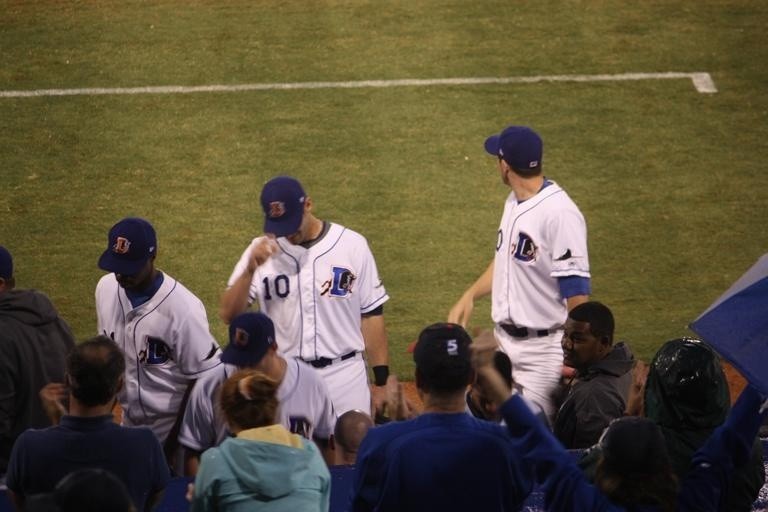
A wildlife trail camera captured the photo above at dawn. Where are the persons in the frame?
[328,409,377,510]
[354,322,583,510]
[0,247,75,512]
[0,337,197,512]
[187,313,336,470]
[183,376,330,511]
[554,301,767,512]
[223,174,391,420]
[94,217,225,474]
[449,126,590,429]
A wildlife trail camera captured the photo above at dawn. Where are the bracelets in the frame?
[371,365,390,387]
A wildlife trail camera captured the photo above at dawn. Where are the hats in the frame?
[483,126,545,168]
[602,417,669,476]
[259,176,307,237]
[97,217,157,275]
[406,322,473,365]
[218,312,276,369]
